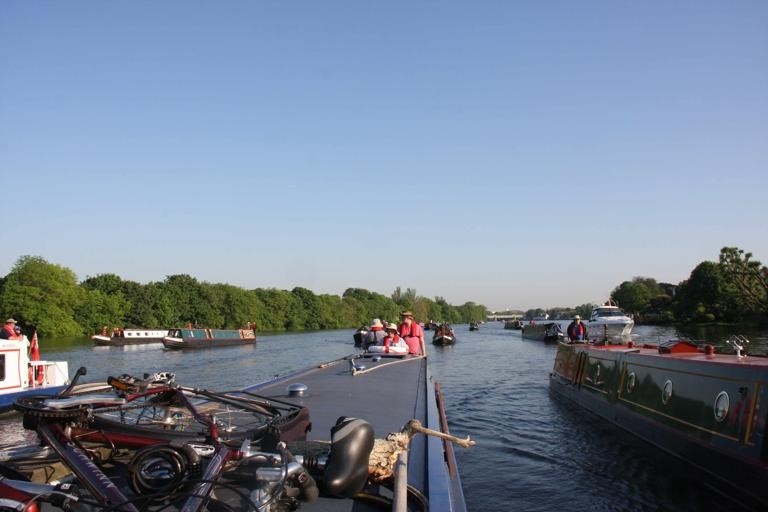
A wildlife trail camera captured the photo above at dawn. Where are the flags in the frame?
[31,332,40,361]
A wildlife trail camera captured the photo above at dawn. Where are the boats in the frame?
[521,322,563,342]
[589,298,635,338]
[0,338,70,409]
[352,317,523,347]
[91,325,169,346]
[549,325,768,512]
[0,348,468,512]
[162,321,257,348]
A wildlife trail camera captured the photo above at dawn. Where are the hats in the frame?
[385,322,400,335]
[399,310,415,317]
[370,319,384,328]
[6,319,17,323]
[571,315,582,319]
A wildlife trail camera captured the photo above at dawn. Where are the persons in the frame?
[0,318,23,341]
[429,319,452,331]
[241,319,256,330]
[567,314,588,343]
[361,309,426,357]
[101,325,108,336]
[14,325,30,347]
[113,327,121,336]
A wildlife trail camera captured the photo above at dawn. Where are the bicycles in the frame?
[0,364,375,512]
